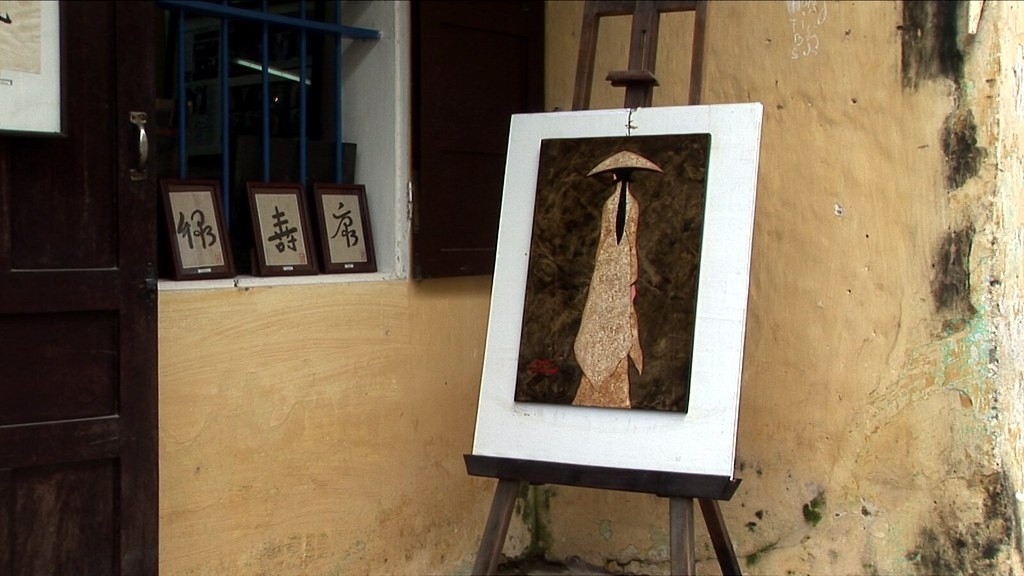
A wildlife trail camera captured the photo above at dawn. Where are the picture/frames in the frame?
[312,185,378,275]
[164,181,234,281]
[1,0,65,139]
[244,181,317,274]
